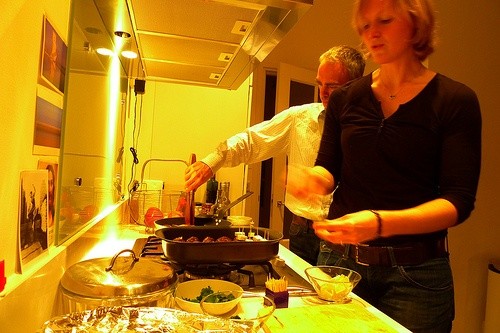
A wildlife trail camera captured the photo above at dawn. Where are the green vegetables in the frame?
[181,286,236,303]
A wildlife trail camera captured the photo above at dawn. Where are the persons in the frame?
[288,1,482,333]
[185,46,365,267]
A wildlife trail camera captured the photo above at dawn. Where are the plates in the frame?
[29,307,247,333]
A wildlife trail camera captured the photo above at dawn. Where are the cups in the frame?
[228,215,252,228]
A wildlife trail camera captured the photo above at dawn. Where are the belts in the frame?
[292,215,314,229]
[320,238,446,267]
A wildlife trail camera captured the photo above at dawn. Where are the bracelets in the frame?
[369,209,382,235]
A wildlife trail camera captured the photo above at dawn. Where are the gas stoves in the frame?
[131,237,319,298]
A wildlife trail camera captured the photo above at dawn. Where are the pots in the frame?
[154,228,283,261]
[152,190,253,229]
[57,248,174,317]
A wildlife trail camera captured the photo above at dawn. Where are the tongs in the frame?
[184,153,197,225]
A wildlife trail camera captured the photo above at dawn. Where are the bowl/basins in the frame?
[174,278,243,314]
[304,265,362,302]
[199,290,275,333]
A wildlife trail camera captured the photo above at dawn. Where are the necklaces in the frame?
[378,76,401,98]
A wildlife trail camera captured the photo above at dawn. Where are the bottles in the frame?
[203,172,230,219]
